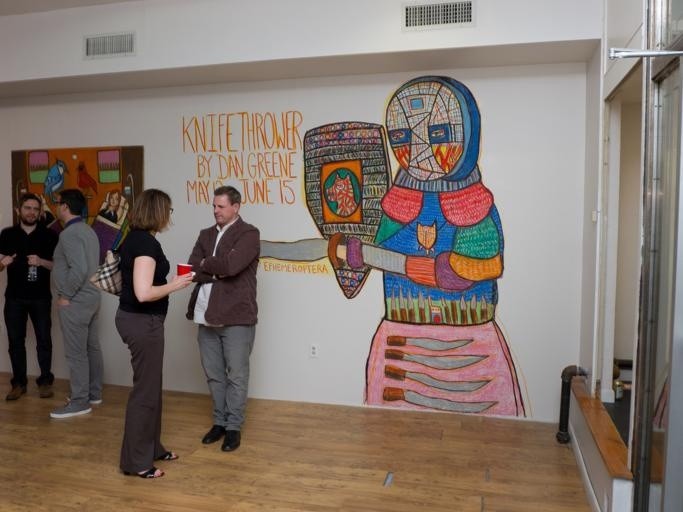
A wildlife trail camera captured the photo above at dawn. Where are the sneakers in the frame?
[48,398,92,419]
[5,384,27,401]
[63,390,102,403]
[37,384,53,398]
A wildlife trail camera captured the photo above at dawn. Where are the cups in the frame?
[176,263,192,276]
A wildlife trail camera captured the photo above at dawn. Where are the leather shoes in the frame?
[220,428,242,452]
[201,423,224,446]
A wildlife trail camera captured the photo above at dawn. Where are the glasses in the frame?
[53,200,65,206]
[168,207,174,215]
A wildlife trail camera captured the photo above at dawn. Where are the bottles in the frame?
[27,263,38,282]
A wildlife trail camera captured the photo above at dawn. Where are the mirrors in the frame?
[590,56,647,470]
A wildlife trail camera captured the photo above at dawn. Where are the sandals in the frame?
[122,466,168,478]
[156,451,179,461]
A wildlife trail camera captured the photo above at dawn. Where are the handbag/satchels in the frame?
[87,246,124,298]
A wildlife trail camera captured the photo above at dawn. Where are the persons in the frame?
[49,188,102,419]
[0,192,59,400]
[114,188,193,479]
[185,185,260,451]
[100,189,120,224]
